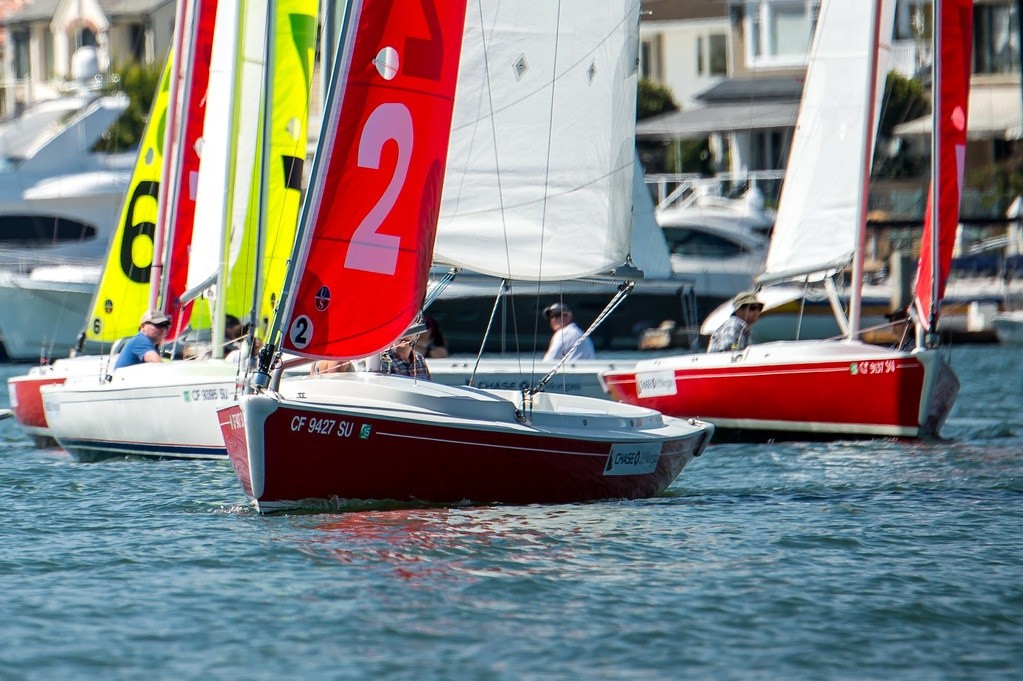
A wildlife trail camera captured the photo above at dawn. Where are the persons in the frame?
[542,303,596,359]
[707,291,764,352]
[415,312,448,358]
[198,312,263,361]
[381,314,432,381]
[114,310,171,369]
[310,359,356,376]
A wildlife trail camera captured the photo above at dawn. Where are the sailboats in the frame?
[0,0,1001,514]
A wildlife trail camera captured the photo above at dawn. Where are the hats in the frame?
[544,302,570,320]
[142,310,171,326]
[884,308,907,318]
[731,291,765,316]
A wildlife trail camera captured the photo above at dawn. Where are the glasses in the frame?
[150,323,168,329]
[889,317,903,323]
[550,313,567,321]
[741,305,761,312]
